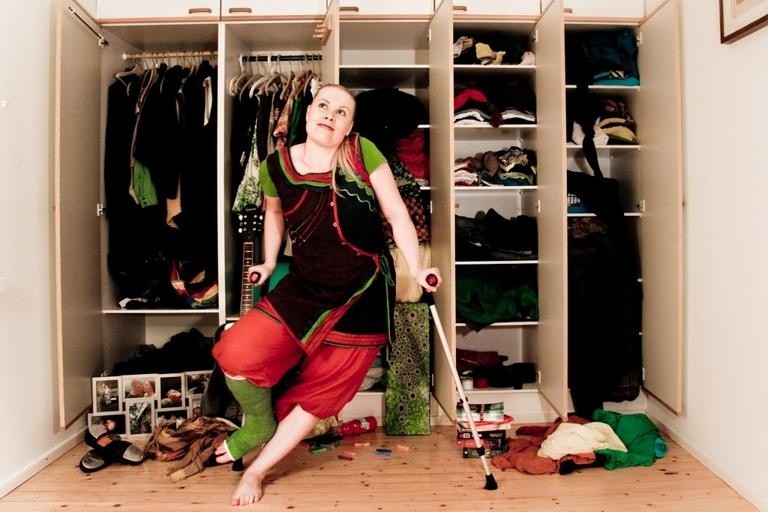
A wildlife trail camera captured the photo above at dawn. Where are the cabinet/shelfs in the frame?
[51,1,687,443]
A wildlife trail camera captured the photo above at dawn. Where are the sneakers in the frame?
[81,425,146,472]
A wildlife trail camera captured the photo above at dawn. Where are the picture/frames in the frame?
[715,0,768,46]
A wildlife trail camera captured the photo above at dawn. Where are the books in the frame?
[455,402,514,458]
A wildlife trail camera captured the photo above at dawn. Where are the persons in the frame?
[197,376,208,393]
[127,379,156,399]
[156,415,184,433]
[101,416,125,435]
[215,83,442,506]
[100,383,111,405]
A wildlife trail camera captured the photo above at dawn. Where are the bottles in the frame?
[340,415,378,436]
